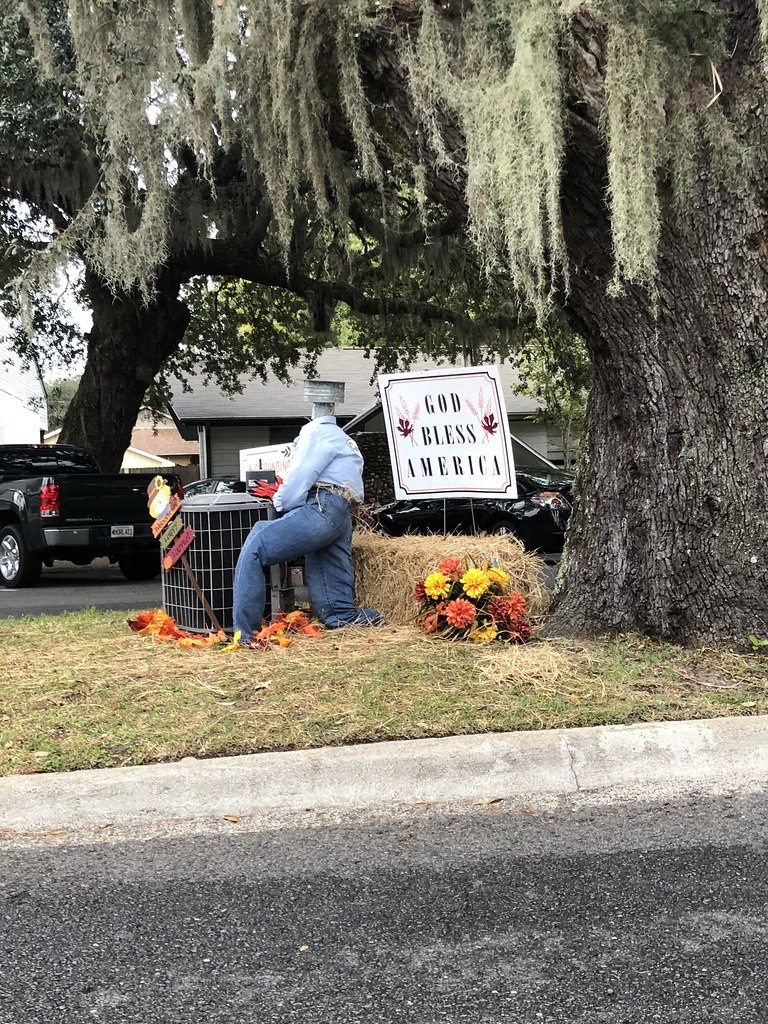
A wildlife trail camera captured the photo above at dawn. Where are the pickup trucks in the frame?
[0,444,185,589]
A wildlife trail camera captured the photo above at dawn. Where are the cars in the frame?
[360,471,574,553]
[183,476,247,499]
